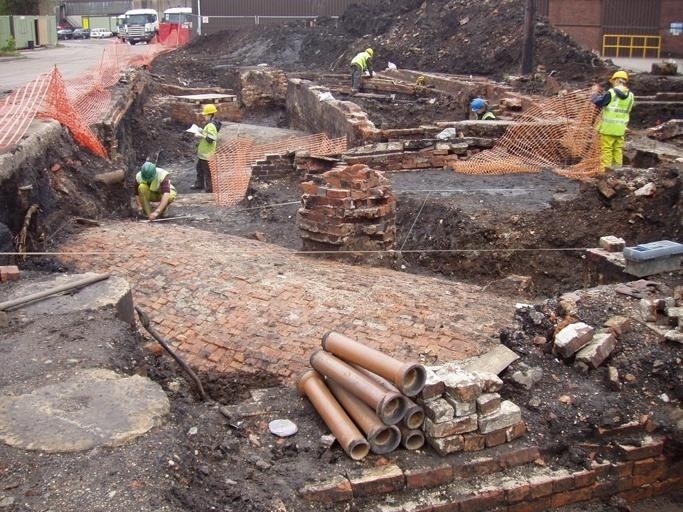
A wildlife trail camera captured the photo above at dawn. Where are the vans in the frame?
[90,28,113,38]
[116,9,159,45]
[162,7,192,29]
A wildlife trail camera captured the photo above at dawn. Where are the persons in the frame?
[350,48,374,92]
[590,70,634,173]
[471,97,496,120]
[190,104,217,194]
[133,162,177,222]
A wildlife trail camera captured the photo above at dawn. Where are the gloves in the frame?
[592,84,599,96]
[194,127,206,140]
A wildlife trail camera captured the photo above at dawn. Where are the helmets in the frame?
[612,71,628,82]
[202,104,217,114]
[141,162,156,181]
[471,98,485,111]
[366,48,373,55]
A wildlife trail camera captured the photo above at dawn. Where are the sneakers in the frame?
[161,207,168,217]
[190,184,206,193]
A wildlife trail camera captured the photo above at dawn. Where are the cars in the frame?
[58,29,90,40]
